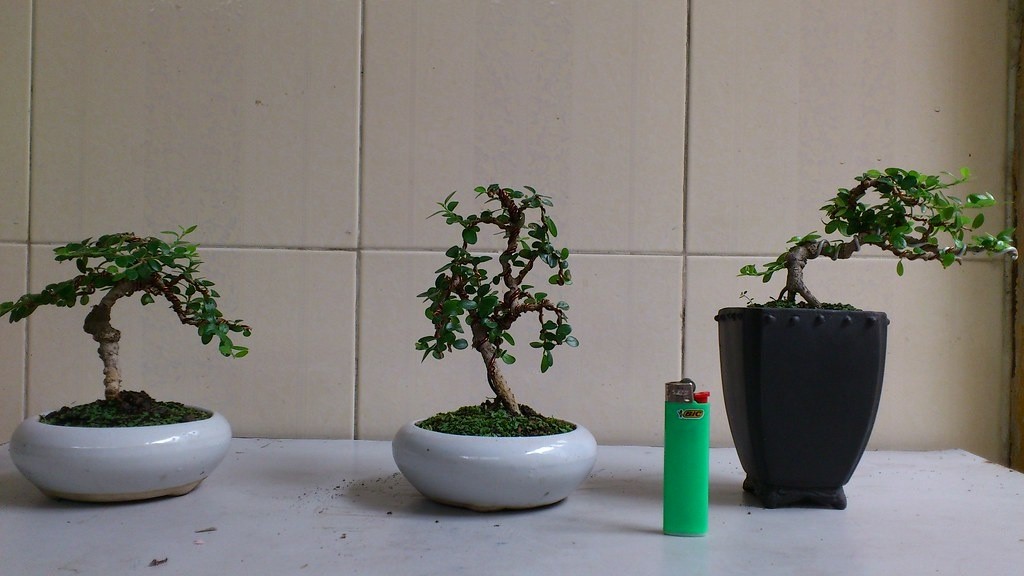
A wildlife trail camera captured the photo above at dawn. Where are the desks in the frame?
[0,439,1024,576]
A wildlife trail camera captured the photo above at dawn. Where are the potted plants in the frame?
[391,183,597,511]
[0,224,253,503]
[714,167,1018,509]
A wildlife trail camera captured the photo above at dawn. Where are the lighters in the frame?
[663,379,710,537]
